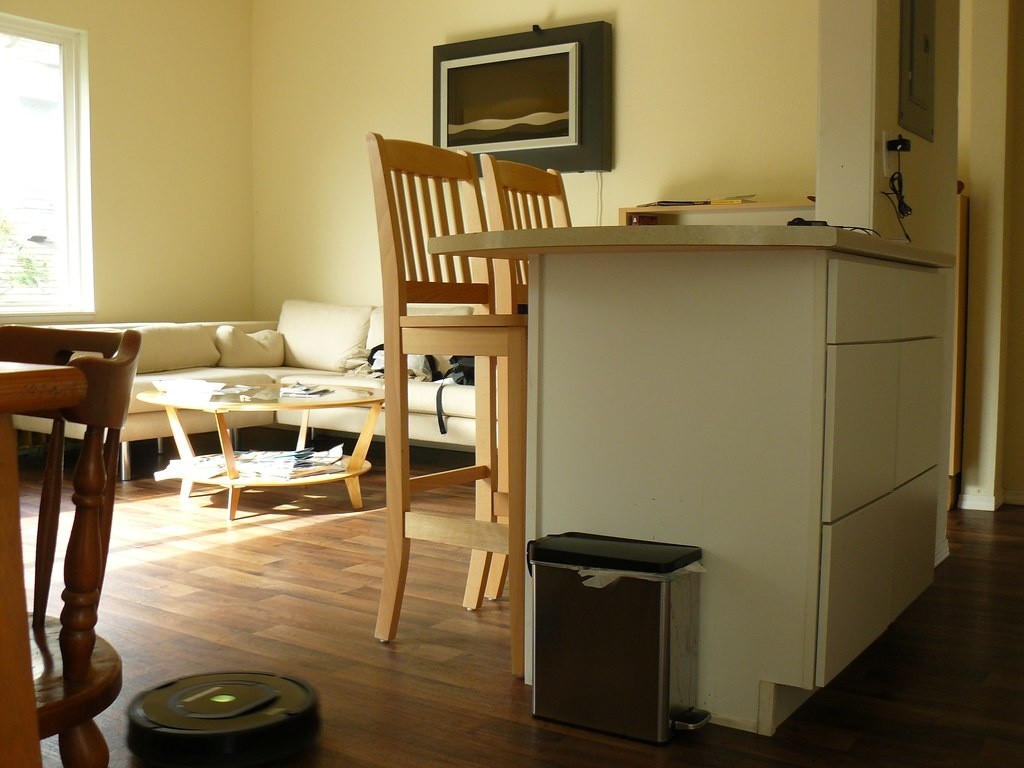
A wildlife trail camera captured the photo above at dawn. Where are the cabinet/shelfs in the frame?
[427,225,959,743]
[617,198,816,225]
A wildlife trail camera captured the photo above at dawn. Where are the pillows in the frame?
[123,324,221,375]
[276,299,373,375]
[213,324,285,367]
[365,305,473,378]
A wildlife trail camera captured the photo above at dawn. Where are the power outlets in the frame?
[883,130,907,183]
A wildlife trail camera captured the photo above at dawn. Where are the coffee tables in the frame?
[136,380,384,519]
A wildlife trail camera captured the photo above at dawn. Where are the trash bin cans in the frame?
[527,531,713,746]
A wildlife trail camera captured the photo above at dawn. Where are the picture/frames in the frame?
[438,41,581,153]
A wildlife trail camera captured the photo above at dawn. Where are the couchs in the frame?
[9,299,504,482]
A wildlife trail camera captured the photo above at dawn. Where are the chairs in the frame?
[476,152,577,599]
[0,322,143,768]
[361,131,528,681]
